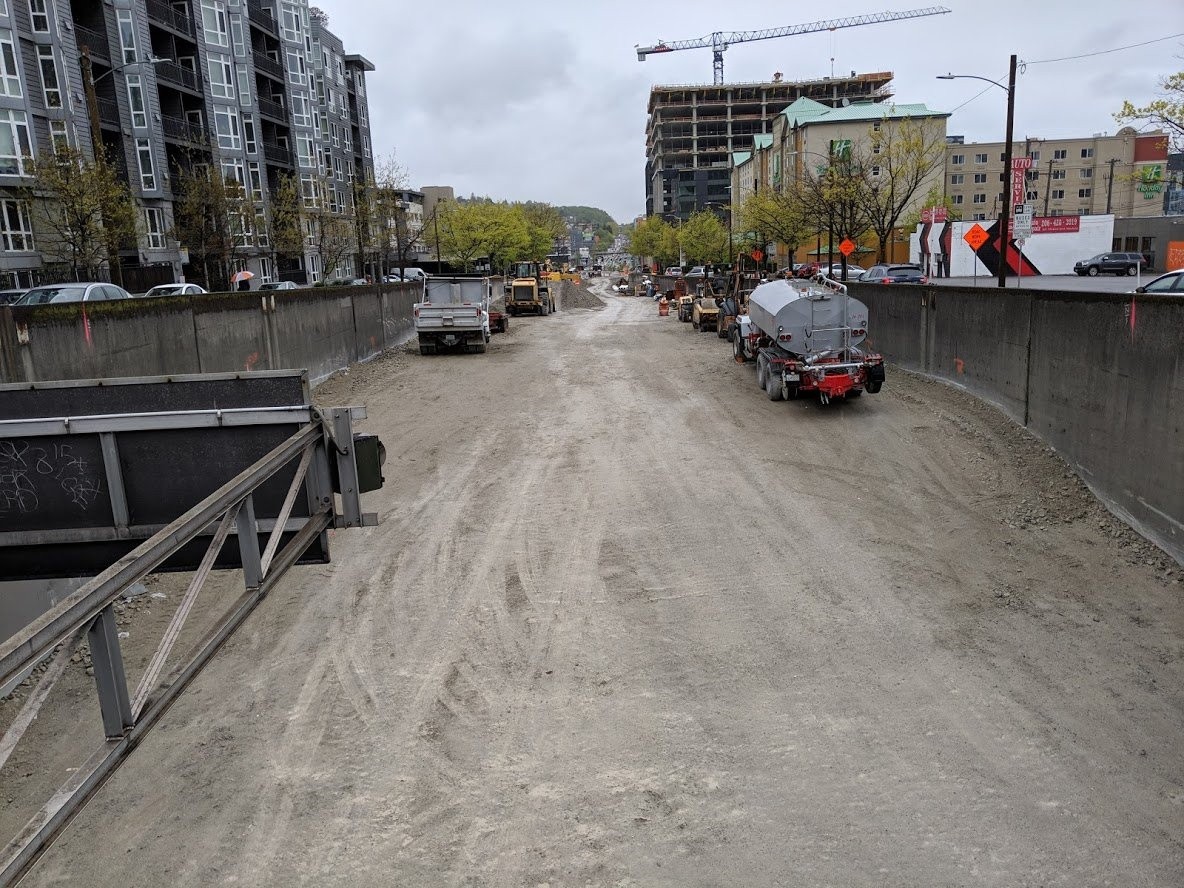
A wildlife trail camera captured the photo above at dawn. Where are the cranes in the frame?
[634,6,952,85]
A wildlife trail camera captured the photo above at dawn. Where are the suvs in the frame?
[859,262,928,286]
[1073,252,1148,277]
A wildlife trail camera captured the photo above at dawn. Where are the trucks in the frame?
[413,273,494,354]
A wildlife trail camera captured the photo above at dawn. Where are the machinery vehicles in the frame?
[503,260,556,317]
[530,253,581,286]
[616,263,886,407]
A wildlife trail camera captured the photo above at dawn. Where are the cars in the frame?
[0,288,55,305]
[326,267,428,286]
[12,281,135,305]
[255,280,300,290]
[141,282,208,297]
[1124,268,1184,296]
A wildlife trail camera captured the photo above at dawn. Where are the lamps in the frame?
[1172,222,1178,224]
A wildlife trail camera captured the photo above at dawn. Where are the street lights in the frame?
[665,214,682,268]
[723,185,759,272]
[785,151,834,281]
[705,201,733,264]
[77,44,174,288]
[934,73,1017,287]
